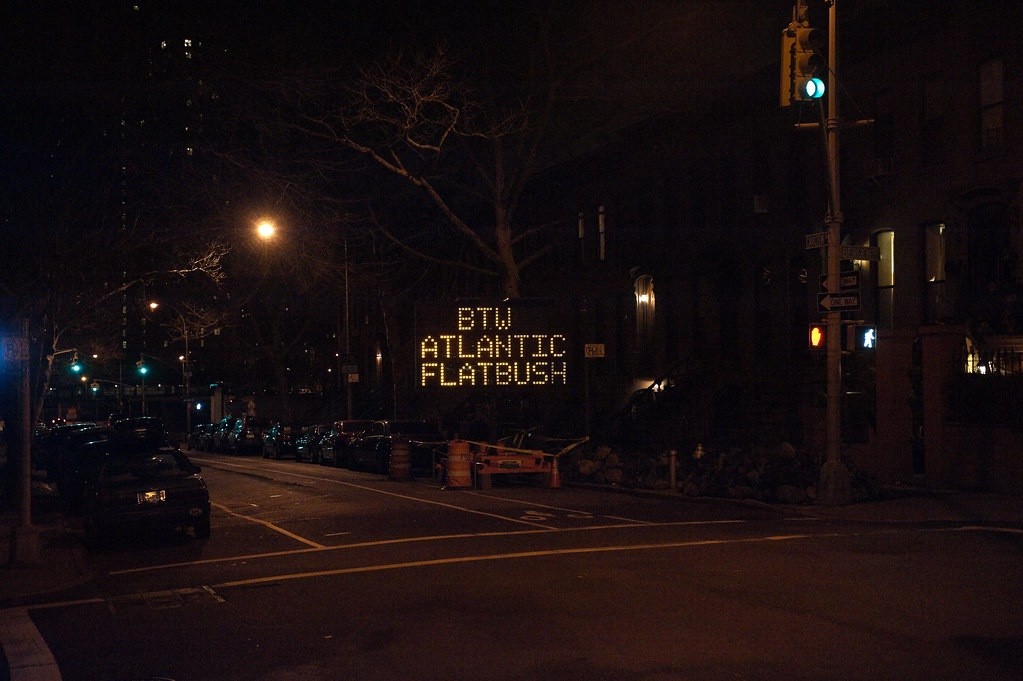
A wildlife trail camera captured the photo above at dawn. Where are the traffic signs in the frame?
[815,273,860,291]
[817,292,859,310]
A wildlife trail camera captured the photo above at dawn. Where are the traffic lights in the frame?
[90,383,99,392]
[789,27,826,104]
[846,325,877,351]
[808,322,828,352]
[138,365,149,377]
[70,363,80,373]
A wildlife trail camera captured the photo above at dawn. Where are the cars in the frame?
[319,419,373,467]
[211,416,235,453]
[348,418,447,476]
[29,412,211,552]
[263,422,296,459]
[294,424,332,464]
[198,423,218,451]
[186,424,205,451]
[226,416,263,454]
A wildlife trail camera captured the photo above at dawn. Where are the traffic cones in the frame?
[547,456,565,489]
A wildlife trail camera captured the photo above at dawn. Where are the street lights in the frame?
[178,355,185,397]
[248,213,351,420]
[146,300,190,435]
